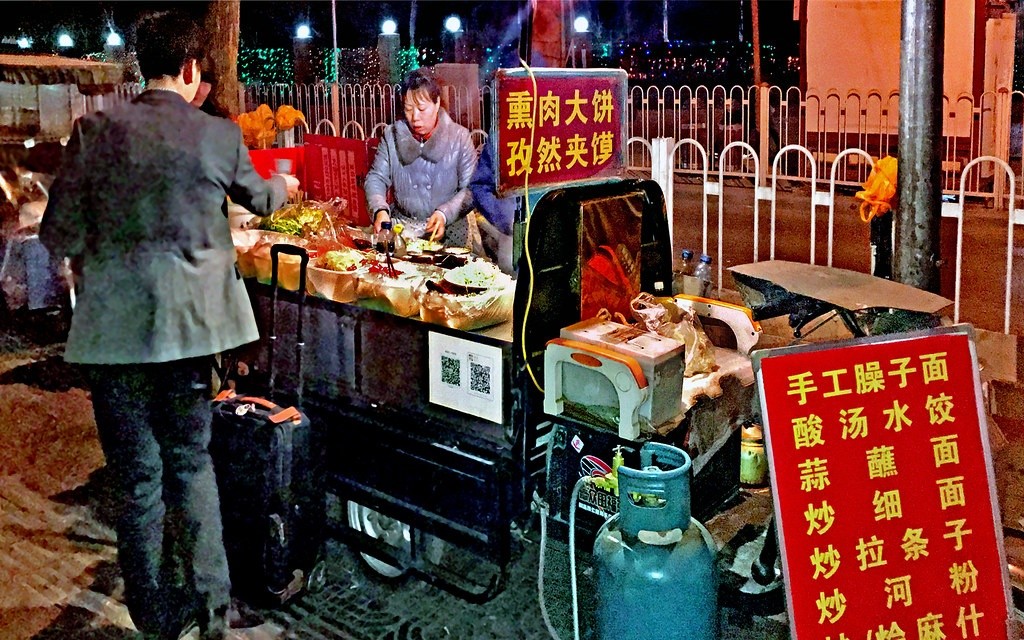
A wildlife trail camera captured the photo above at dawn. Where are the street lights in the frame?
[377,19,401,85]
[57,33,75,58]
[441,16,466,63]
[103,32,125,54]
[292,25,314,83]
[572,16,594,66]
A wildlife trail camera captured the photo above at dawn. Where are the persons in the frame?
[39,12,299,640]
[468,122,516,263]
[365,69,478,248]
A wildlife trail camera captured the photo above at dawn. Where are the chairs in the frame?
[523,177,763,440]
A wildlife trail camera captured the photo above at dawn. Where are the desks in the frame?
[727,259,955,338]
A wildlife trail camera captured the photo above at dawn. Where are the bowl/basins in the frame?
[418,247,495,298]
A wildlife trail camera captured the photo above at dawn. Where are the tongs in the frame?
[385,251,399,279]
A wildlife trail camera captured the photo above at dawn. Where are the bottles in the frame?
[377,221,396,258]
[388,223,406,258]
[674,248,696,276]
[690,254,713,299]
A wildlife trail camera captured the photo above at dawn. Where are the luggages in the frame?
[212,244,330,605]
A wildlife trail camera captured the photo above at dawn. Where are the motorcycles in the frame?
[207,176,1024,618]
[0,58,125,331]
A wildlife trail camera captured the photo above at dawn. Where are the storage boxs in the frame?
[560,318,686,427]
